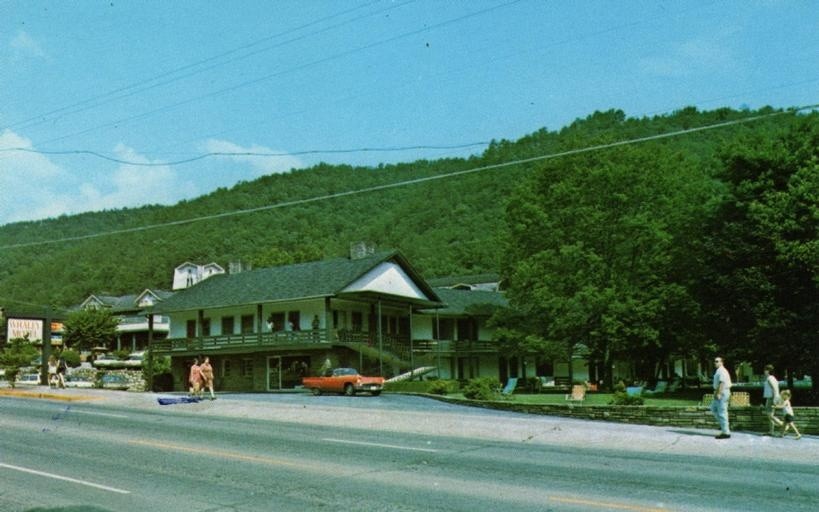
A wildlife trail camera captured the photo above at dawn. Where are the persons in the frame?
[763,364,790,436]
[711,356,731,438]
[47,355,59,388]
[266,317,273,342]
[312,316,320,343]
[771,389,801,439]
[288,320,294,341]
[57,357,69,388]
[199,356,216,399]
[188,357,207,398]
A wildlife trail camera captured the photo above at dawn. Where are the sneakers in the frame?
[794,434,801,440]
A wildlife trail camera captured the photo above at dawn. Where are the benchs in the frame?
[536,377,573,394]
[506,378,535,393]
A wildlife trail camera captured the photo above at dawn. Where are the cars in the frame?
[302,367,384,396]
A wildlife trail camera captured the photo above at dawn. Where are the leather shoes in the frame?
[716,434,730,439]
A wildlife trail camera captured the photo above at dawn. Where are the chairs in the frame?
[566,384,587,405]
